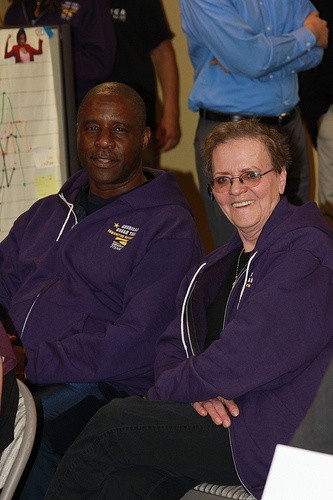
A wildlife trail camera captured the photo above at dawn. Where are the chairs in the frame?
[0,380,37,500]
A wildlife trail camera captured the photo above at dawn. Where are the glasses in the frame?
[209,168,274,193]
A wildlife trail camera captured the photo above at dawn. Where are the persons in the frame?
[42,119,333,500]
[0,83,209,500]
[4,0,182,169]
[177,0,333,249]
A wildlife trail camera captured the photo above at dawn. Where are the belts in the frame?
[199,109,297,127]
